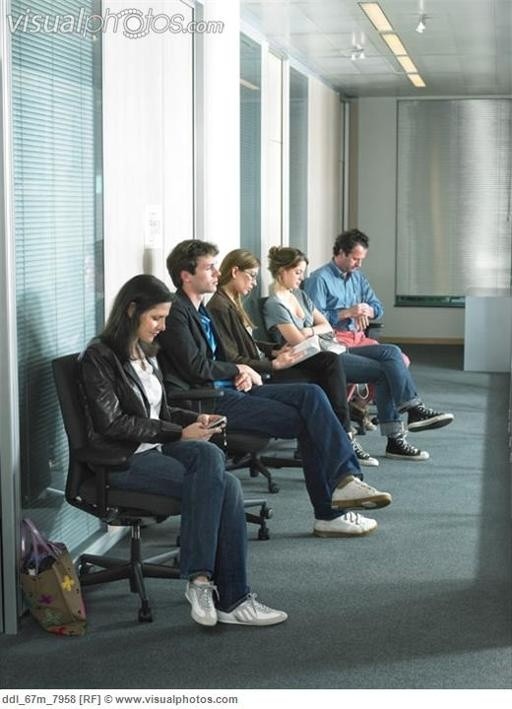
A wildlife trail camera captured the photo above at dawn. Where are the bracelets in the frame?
[311,327,315,335]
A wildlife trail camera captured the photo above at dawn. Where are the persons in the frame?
[75,275,287,626]
[263,247,454,460]
[157,240,391,538]
[204,250,379,466]
[304,229,410,431]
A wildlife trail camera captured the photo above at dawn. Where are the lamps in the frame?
[414,14,427,35]
[352,47,365,61]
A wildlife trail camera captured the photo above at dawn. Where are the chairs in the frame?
[300,281,385,425]
[51,352,186,623]
[258,297,367,436]
[157,350,274,541]
[225,342,303,493]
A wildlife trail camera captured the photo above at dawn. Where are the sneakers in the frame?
[216,598,287,625]
[349,437,378,467]
[361,416,376,429]
[384,436,430,460]
[313,512,378,539]
[407,404,454,432]
[331,480,392,509]
[185,580,218,626]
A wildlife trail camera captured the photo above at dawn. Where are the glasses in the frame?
[242,270,260,280]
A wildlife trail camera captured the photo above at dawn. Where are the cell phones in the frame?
[208,416,227,429]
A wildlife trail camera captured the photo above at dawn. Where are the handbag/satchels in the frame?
[19,517,86,637]
